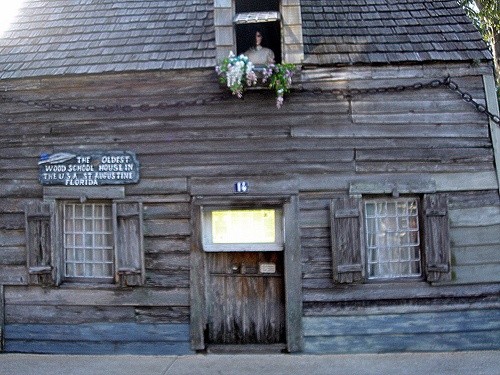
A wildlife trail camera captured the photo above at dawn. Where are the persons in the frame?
[238,28,275,66]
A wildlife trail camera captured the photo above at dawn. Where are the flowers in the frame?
[260,63,297,110]
[215,50,257,98]
